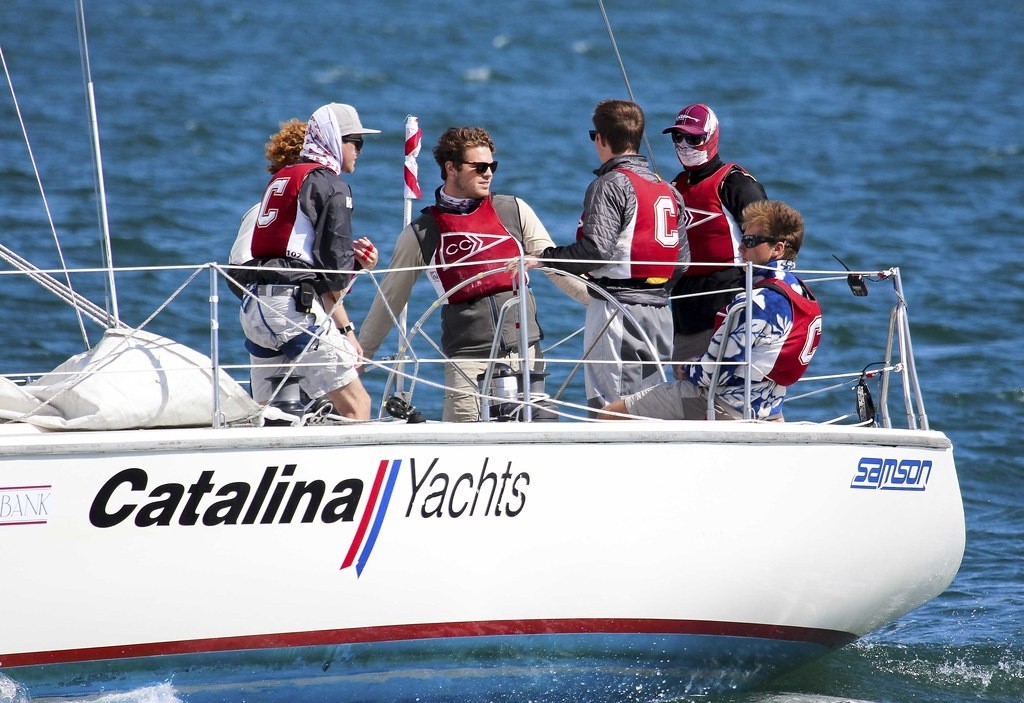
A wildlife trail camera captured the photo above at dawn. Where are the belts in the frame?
[243,285,301,296]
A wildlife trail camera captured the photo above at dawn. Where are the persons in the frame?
[226,102,382,425]
[356,127,590,423]
[596,199,821,420]
[504,99,690,421]
[662,103,769,370]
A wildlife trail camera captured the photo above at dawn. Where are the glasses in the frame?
[589,130,597,141]
[342,137,363,151]
[462,160,499,174]
[740,233,790,248]
[672,130,706,145]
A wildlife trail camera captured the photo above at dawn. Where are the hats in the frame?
[662,103,720,135]
[311,101,381,136]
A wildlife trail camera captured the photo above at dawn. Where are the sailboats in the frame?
[0,1,968,703]
